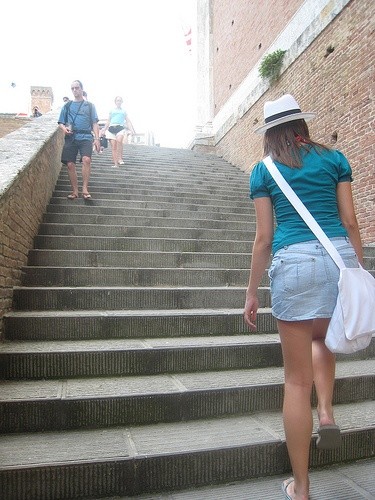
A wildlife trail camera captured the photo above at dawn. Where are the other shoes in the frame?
[112,166,119,169]
[118,160,124,164]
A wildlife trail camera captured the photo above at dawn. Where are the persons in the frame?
[63,91,136,169]
[33,109,42,117]
[244,94,364,500]
[58,80,100,200]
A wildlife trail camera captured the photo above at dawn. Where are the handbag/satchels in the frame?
[64,135,75,144]
[325,268,375,354]
[100,135,107,148]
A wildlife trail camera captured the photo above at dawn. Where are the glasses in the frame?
[72,87,79,89]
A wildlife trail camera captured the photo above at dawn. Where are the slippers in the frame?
[67,194,79,199]
[82,193,92,200]
[281,477,294,500]
[316,424,341,449]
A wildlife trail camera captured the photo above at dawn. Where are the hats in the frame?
[254,94,316,133]
